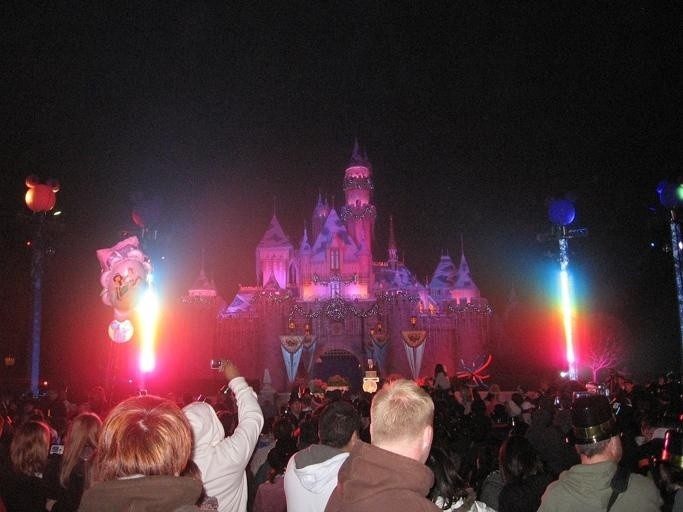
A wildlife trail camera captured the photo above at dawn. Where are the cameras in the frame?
[210,359,227,370]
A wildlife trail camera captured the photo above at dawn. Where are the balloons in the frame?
[93,234,150,324]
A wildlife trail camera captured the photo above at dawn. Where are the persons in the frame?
[2,353,682,512]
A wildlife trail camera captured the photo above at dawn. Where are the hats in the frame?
[287,394,301,403]
[657,431,682,470]
[565,395,632,445]
